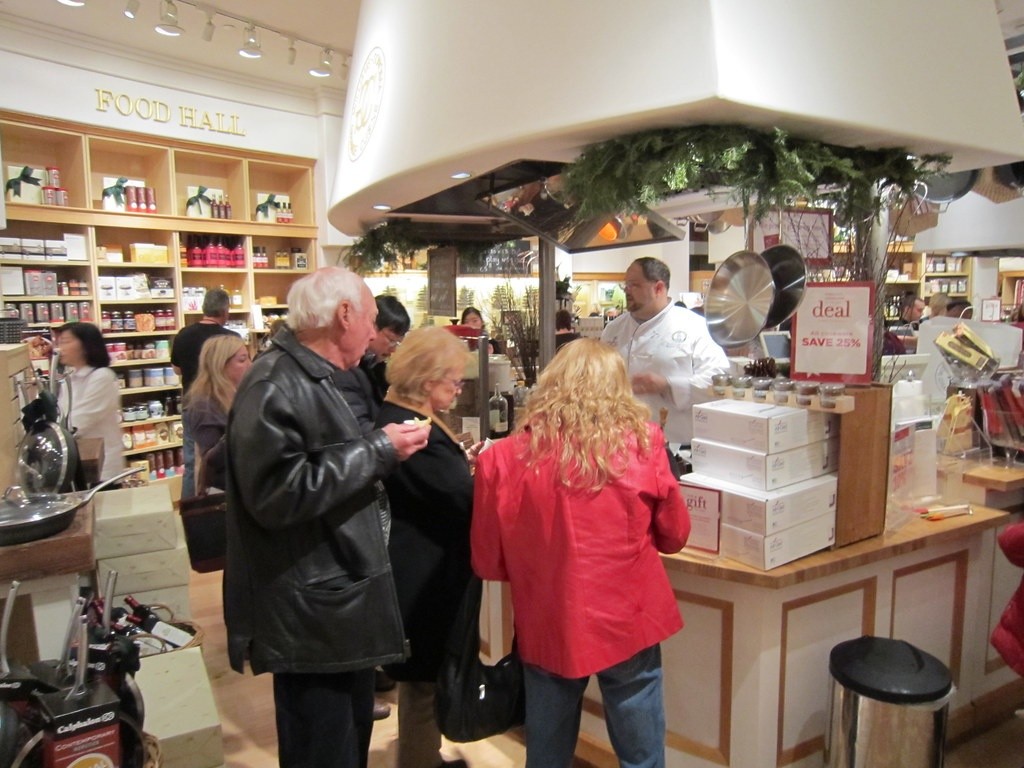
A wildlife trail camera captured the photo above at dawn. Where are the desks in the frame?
[74,436,104,486]
[0,489,93,577]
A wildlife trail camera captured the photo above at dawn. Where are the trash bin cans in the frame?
[827,634,957,768]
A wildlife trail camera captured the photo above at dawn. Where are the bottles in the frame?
[65,586,194,668]
[253,246,289,269]
[884,296,901,316]
[210,193,232,219]
[178,233,245,268]
[488,384,508,434]
[77,309,187,479]
[231,285,242,308]
[219,285,231,309]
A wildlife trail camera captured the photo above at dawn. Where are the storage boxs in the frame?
[89,482,192,621]
[935,323,1002,378]
[10,644,226,768]
[670,400,841,573]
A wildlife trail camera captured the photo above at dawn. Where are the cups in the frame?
[709,374,846,409]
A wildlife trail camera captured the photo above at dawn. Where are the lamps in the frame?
[60,0,351,78]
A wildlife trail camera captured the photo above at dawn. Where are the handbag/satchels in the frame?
[179,492,226,574]
[432,569,526,743]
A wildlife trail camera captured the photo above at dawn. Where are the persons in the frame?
[171,256,733,768]
[990,522,1024,720]
[52,322,128,491]
[883,291,973,355]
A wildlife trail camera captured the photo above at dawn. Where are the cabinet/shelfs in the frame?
[831,242,1023,325]
[0,107,316,506]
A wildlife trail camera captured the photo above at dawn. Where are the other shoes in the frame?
[442,757,466,768]
[371,667,396,719]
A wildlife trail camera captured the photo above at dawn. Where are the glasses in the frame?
[441,373,466,391]
[379,329,405,348]
[619,280,656,291]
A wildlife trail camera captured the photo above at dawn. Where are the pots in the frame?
[703,188,774,348]
[751,191,809,333]
[0,466,148,547]
